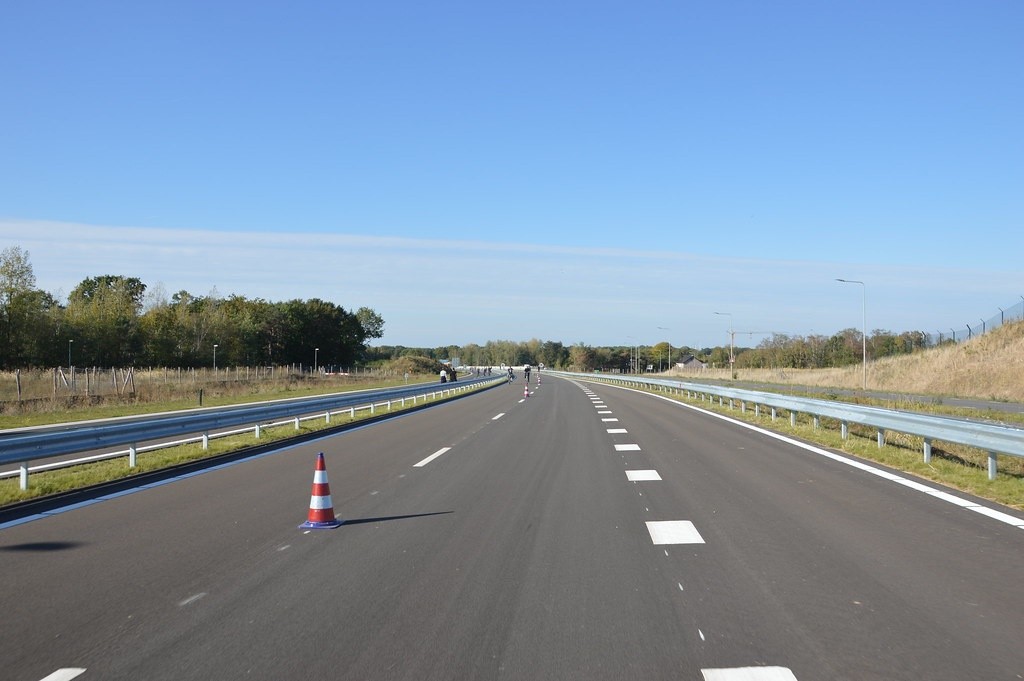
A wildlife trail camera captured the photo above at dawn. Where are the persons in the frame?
[450,368,457,382]
[524,365,531,382]
[507,365,514,382]
[476,366,493,376]
[440,368,447,384]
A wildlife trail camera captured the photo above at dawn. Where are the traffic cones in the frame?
[522,382,531,398]
[299,453,344,529]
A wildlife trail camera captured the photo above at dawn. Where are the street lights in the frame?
[654,326,671,371]
[68,340,74,372]
[714,311,735,383]
[834,278,866,387]
[213,345,220,372]
[314,347,319,373]
